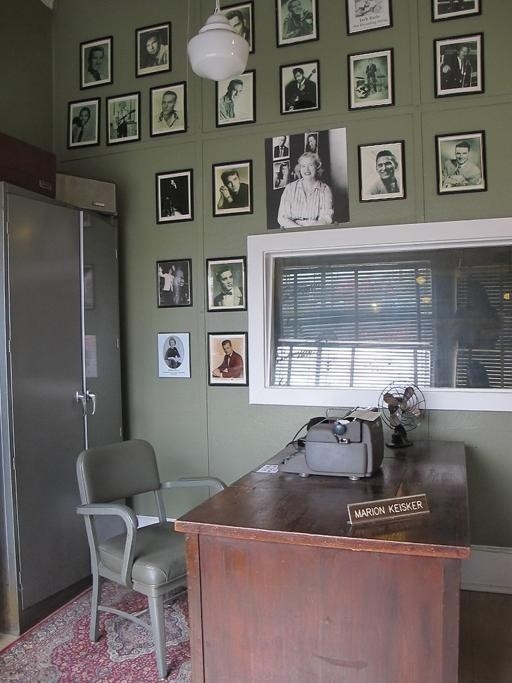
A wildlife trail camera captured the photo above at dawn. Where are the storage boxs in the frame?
[0,133,56,198]
[56,173,117,215]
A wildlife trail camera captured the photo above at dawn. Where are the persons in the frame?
[155,264,186,301]
[277,152,335,228]
[157,91,182,132]
[287,68,316,108]
[212,164,250,210]
[85,45,106,81]
[163,338,181,370]
[442,141,481,189]
[213,339,243,377]
[116,100,132,138]
[165,178,182,219]
[368,151,400,195]
[439,43,472,86]
[214,268,242,307]
[280,1,312,38]
[139,34,167,67]
[218,78,251,123]
[225,7,249,45]
[365,58,376,93]
[270,135,292,155]
[271,162,290,189]
[74,104,94,140]
[303,132,316,152]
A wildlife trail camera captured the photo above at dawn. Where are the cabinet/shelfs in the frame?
[0,182,123,636]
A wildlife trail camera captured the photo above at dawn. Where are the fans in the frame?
[378,381,426,448]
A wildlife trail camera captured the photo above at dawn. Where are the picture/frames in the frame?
[155,168,193,224]
[280,60,320,115]
[156,259,193,307]
[348,48,394,110]
[135,21,171,78]
[207,331,248,386]
[431,0,481,22]
[106,91,141,146]
[67,97,100,150]
[358,140,407,203]
[345,0,393,35]
[80,35,112,90]
[212,159,253,216]
[433,32,485,98]
[275,0,319,47]
[206,256,247,312]
[215,69,256,128]
[220,1,254,53]
[150,81,186,137]
[435,130,488,195]
[156,332,191,379]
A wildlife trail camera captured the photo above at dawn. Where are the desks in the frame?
[174,440,470,682]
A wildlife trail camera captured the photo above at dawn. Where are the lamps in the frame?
[187,0,249,81]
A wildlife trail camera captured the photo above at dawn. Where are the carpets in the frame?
[0,578,191,683]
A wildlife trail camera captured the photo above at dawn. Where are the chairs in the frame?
[76,439,227,677]
